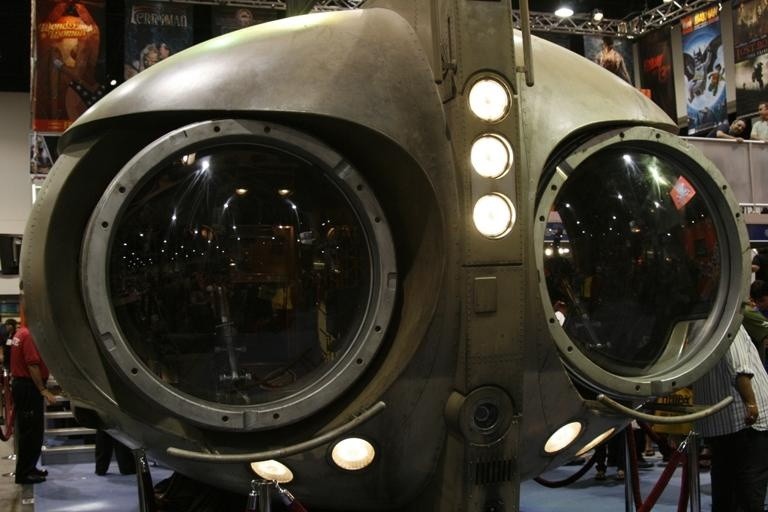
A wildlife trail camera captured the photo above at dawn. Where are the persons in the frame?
[594,35,632,83]
[50,1,112,120]
[1,309,52,487]
[534,361,535,386]
[90,425,137,477]
[597,280,768,512]
[704,115,747,142]
[747,102,768,141]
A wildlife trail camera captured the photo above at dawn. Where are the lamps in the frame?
[588,7,604,26]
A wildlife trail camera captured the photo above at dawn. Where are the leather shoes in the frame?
[31,468,48,477]
[15,475,46,484]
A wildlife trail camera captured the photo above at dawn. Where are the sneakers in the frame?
[638,461,654,468]
[596,470,606,480]
[645,449,654,456]
[617,470,625,480]
